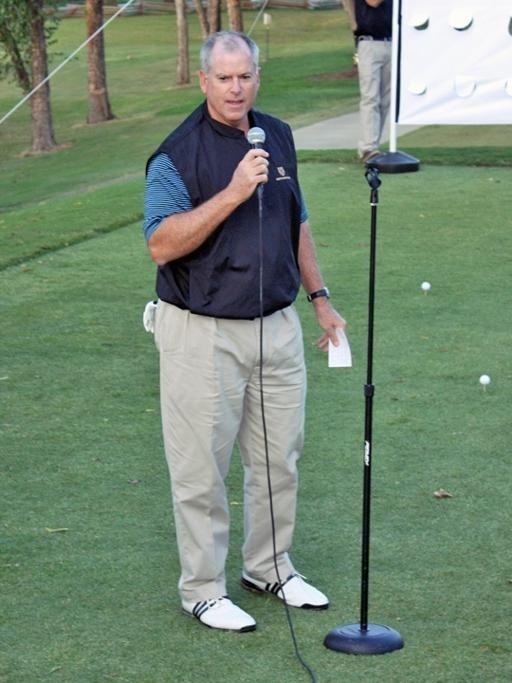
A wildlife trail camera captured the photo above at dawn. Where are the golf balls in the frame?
[479,375,490,384]
[422,282,430,290]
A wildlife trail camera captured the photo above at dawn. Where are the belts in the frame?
[357,35,393,42]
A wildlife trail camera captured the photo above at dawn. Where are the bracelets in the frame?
[306,283,332,302]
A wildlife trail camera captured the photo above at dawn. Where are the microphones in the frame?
[247,127,266,201]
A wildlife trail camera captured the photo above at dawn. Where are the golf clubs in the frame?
[359,153,420,631]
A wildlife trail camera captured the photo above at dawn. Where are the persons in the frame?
[141,26,345,631]
[342,0,394,160]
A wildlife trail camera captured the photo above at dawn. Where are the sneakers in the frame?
[179,593,257,633]
[240,566,332,611]
